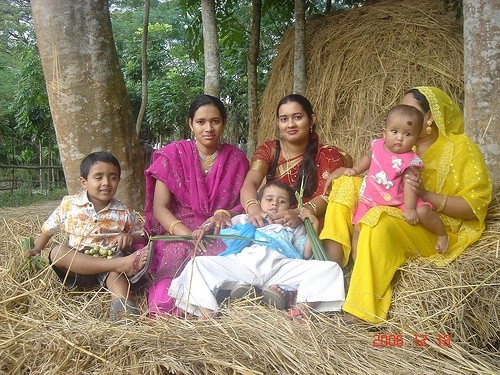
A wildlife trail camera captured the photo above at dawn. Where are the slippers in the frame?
[128,241,154,284]
[109,296,137,325]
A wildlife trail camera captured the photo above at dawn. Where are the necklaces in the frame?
[282,139,298,186]
[197,147,219,174]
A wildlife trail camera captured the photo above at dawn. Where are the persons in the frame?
[344,105,448,264]
[168,180,345,325]
[318,86,493,330]
[142,95,248,317]
[25,152,153,320]
[239,93,346,237]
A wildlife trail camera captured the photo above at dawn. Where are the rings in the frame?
[216,223,220,227]
[264,213,268,219]
[282,216,287,223]
[287,222,290,226]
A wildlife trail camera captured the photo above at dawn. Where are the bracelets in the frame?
[245,199,261,212]
[168,219,181,235]
[213,209,231,217]
[304,201,317,216]
[437,194,447,212]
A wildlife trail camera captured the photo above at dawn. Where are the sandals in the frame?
[230,284,261,301]
[261,284,297,310]
